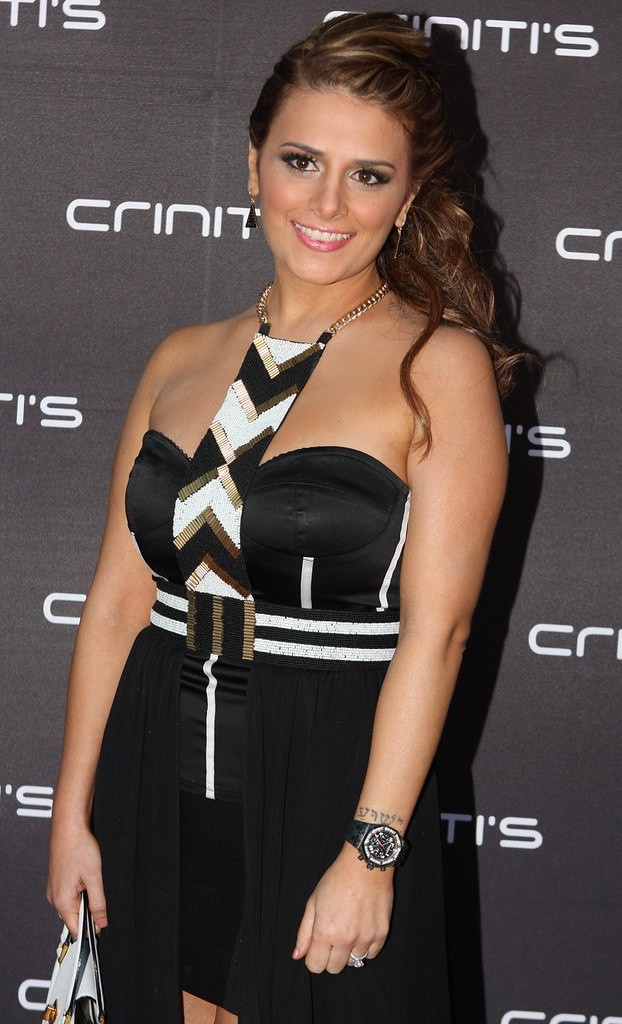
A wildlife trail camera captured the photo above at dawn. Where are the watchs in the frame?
[344,819,408,871]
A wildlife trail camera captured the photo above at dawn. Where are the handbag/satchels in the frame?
[39,890,105,1024]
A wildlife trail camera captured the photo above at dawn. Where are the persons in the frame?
[46,11,510,1024]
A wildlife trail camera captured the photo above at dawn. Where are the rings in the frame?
[349,951,369,968]
